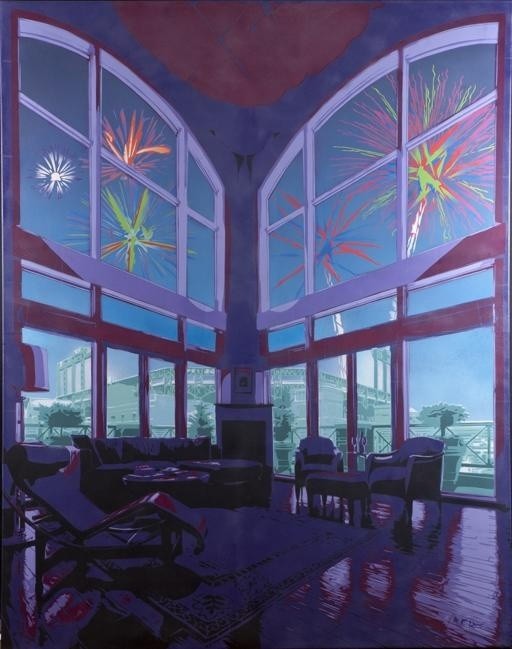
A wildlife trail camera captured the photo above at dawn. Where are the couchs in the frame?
[72,434,272,510]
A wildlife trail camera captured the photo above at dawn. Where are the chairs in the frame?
[293,435,443,519]
[3,443,209,596]
